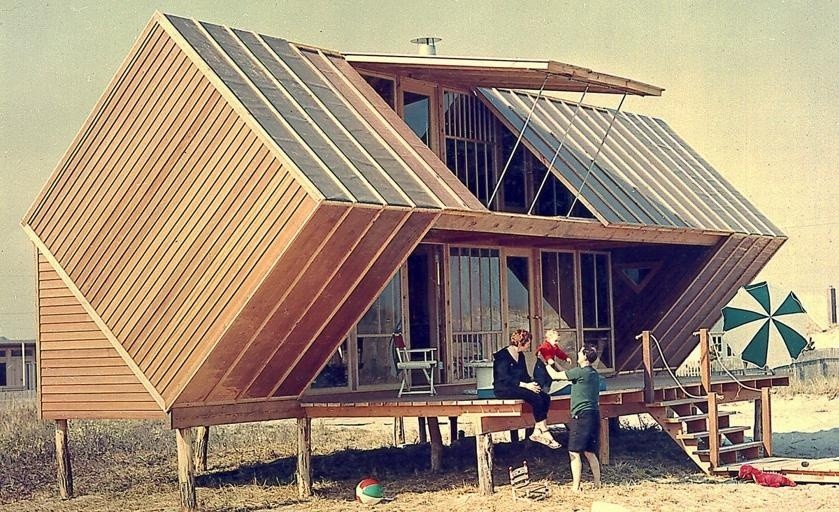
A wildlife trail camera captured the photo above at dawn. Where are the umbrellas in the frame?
[720,280,810,370]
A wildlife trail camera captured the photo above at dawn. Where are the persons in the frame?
[491,327,563,450]
[532,329,572,392]
[537,345,602,491]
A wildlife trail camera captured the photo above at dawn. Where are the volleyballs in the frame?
[356,479,384,506]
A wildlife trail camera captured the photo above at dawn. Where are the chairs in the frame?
[393,332,439,398]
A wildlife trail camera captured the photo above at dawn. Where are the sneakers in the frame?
[529,429,562,449]
